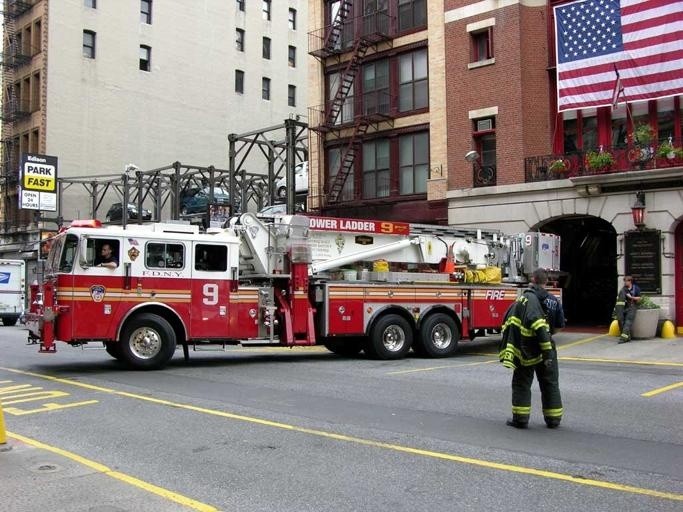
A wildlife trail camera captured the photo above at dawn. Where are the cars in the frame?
[106,203,152,222]
[181,184,240,213]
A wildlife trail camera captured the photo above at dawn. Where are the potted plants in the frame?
[655,139,682,160]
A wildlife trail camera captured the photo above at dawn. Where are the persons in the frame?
[93,244,117,268]
[505,267,566,428]
[616,274,642,343]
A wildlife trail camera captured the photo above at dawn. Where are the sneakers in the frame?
[506,419,528,429]
[546,420,559,429]
[618,337,632,343]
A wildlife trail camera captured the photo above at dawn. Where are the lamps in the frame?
[464,150,488,179]
[630,192,647,227]
[477,119,492,132]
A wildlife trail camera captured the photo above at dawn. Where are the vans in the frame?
[275,160,308,197]
[1,258,26,325]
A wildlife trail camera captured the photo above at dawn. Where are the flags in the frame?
[553,1,682,113]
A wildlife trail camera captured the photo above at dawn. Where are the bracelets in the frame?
[100,263,102,266]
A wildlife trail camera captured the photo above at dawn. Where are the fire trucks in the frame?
[32,204,564,359]
